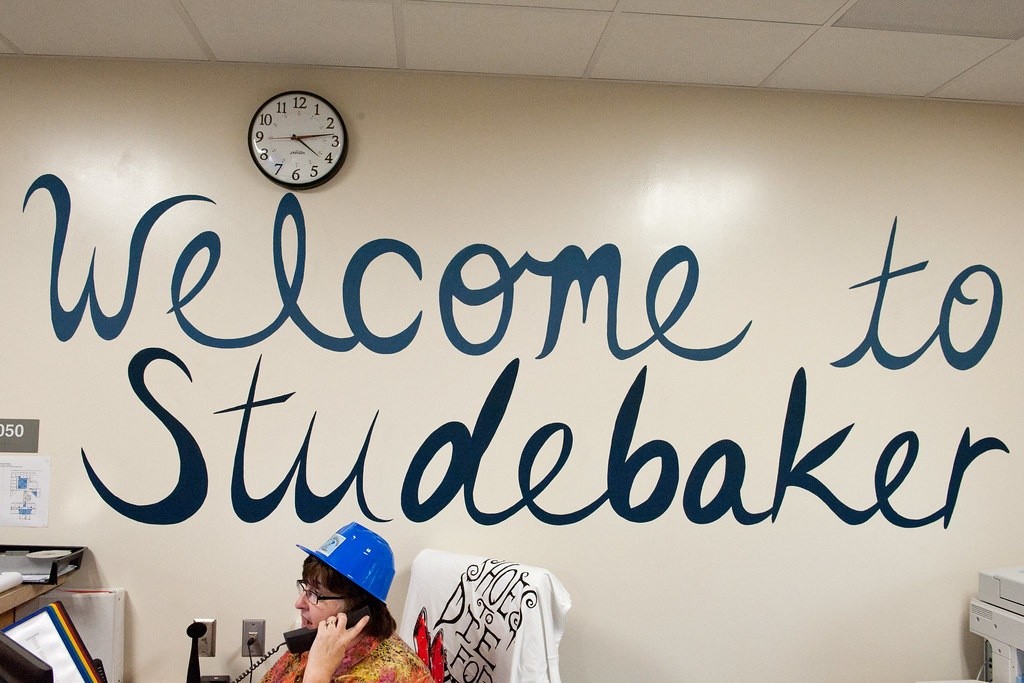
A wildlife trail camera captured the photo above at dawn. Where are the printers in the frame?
[970,569,1024,683]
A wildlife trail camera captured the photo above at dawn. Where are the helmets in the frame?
[294,521,398,603]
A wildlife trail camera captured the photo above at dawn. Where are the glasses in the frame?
[293,578,360,605]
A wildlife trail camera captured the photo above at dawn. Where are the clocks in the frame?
[247,91,348,190]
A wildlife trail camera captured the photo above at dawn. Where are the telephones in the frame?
[282,602,374,655]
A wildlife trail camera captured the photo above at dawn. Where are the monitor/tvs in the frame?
[0,632,54,683]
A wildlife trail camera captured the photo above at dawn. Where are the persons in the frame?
[260,522,438,683]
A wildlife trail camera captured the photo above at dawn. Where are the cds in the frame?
[26,550,71,558]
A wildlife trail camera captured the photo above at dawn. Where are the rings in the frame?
[326,620,336,626]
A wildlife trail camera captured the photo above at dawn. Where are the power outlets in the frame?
[242,619,265,657]
[193,618,216,657]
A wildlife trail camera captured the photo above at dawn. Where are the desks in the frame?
[0,574,66,631]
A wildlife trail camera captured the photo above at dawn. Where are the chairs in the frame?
[398,550,572,683]
